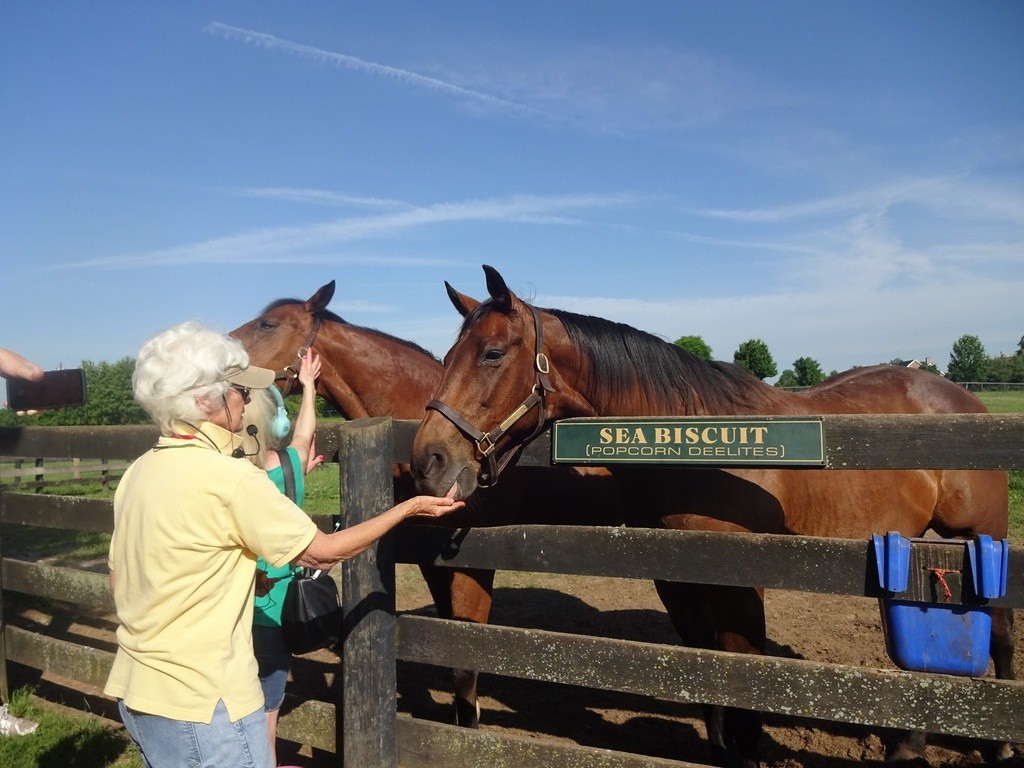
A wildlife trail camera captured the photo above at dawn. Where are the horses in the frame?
[224,263,1015,768]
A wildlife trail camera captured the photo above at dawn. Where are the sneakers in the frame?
[0,703,39,736]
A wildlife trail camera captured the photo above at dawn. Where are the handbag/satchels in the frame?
[281,576,343,656]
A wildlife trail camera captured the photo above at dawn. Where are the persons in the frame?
[102,321,467,768]
[0,348,62,415]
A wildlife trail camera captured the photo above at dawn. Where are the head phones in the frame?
[267,384,291,439]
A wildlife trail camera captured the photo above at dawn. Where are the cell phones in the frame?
[6,368,87,412]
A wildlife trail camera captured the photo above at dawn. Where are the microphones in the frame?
[244,424,261,457]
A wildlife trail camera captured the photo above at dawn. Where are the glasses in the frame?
[226,385,252,402]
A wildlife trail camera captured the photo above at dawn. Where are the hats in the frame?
[180,349,275,389]
